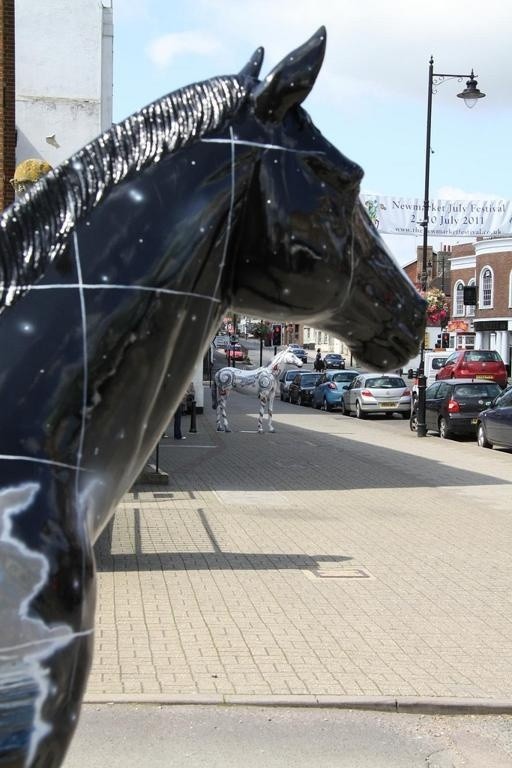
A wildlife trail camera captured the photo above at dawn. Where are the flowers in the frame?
[416,288,449,327]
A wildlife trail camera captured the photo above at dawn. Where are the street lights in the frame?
[415,52,486,438]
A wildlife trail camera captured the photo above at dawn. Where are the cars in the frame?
[214,334,245,361]
[423,348,508,390]
[278,369,412,419]
[409,376,503,439]
[475,385,512,449]
[287,343,307,364]
[323,353,346,370]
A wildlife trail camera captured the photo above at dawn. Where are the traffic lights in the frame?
[434,333,449,348]
[262,323,282,348]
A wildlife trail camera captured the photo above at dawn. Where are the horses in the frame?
[2,17,434,768]
[211,344,305,434]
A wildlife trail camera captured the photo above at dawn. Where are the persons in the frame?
[172,406,186,440]
[313,356,321,373]
[320,359,325,373]
[315,348,321,361]
